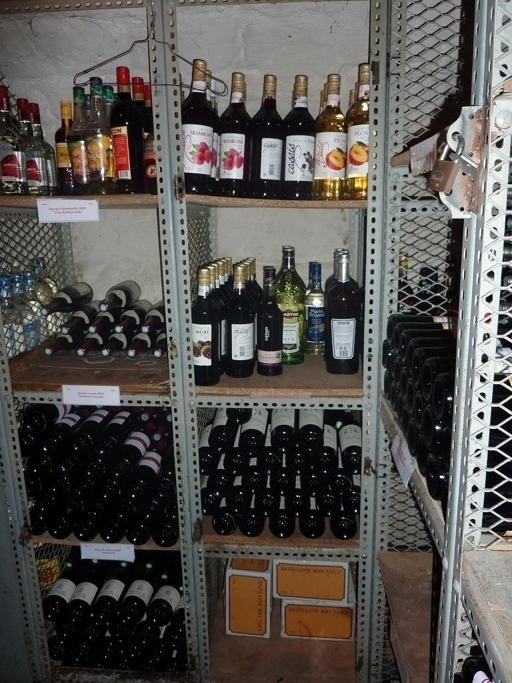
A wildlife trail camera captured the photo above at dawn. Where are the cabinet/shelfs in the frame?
[372,2,512,677]
[163,0,376,618]
[1,3,195,678]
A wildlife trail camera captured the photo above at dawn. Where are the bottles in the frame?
[154,331,171,359]
[305,263,328,356]
[67,86,94,195]
[76,331,109,357]
[348,91,354,111]
[204,70,219,194]
[44,329,83,356]
[181,60,214,192]
[88,311,115,334]
[28,259,57,340]
[0,83,21,194]
[327,254,361,374]
[314,74,346,200]
[99,86,113,119]
[10,274,37,352]
[282,75,315,198]
[323,84,331,109]
[141,84,163,194]
[114,300,153,334]
[348,63,378,200]
[275,248,305,365]
[81,93,93,118]
[98,280,141,313]
[7,95,21,131]
[250,73,285,200]
[38,257,64,334]
[22,271,47,347]
[380,311,512,537]
[189,252,282,386]
[40,281,93,317]
[16,97,50,198]
[131,77,143,121]
[101,331,134,357]
[275,246,306,291]
[42,562,192,675]
[0,277,25,365]
[54,99,79,192]
[126,332,158,358]
[141,300,169,334]
[318,90,327,113]
[354,84,359,106]
[19,403,184,551]
[305,261,323,295]
[109,66,140,191]
[219,72,251,197]
[60,299,101,335]
[83,76,113,194]
[27,103,57,194]
[200,404,363,545]
